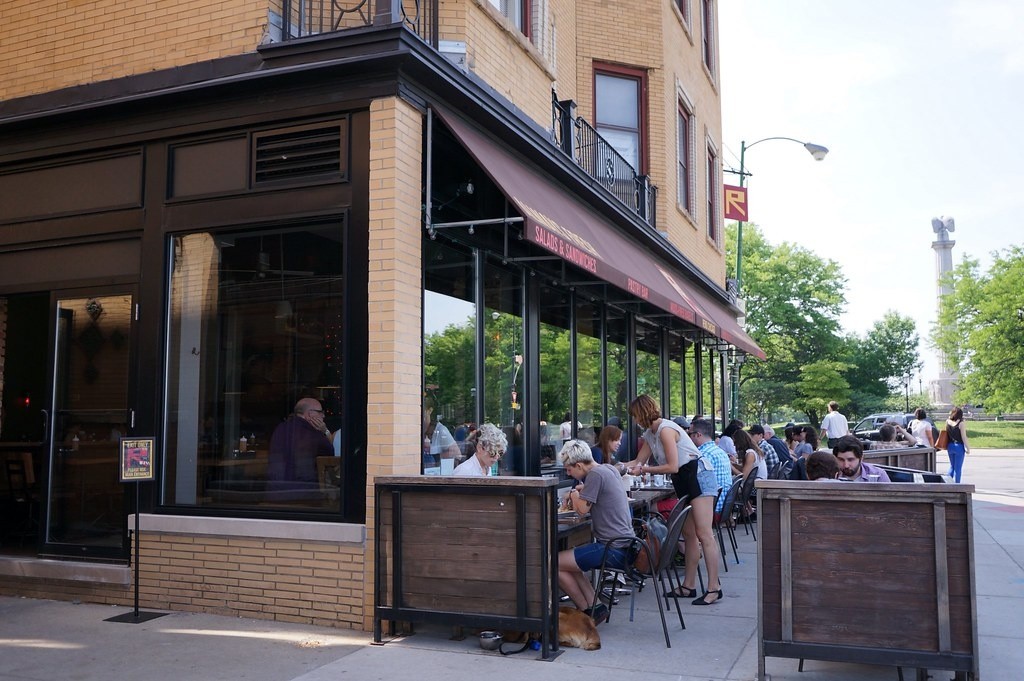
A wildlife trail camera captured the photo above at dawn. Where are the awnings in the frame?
[428,100,766,360]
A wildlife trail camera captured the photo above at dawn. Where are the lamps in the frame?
[271,233,292,317]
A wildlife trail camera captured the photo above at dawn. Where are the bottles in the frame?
[644,473,651,486]
[72,434,79,451]
[250,433,255,445]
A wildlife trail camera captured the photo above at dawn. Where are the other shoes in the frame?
[719,521,736,527]
[582,603,609,626]
[749,510,758,522]
[733,516,750,523]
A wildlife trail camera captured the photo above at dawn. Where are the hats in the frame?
[672,416,690,428]
[608,416,621,426]
[781,423,795,429]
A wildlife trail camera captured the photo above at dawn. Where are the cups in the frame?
[240,436,247,452]
[654,474,664,487]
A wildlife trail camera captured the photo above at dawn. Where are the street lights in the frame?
[755,398,762,426]
[902,373,910,414]
[732,137,829,419]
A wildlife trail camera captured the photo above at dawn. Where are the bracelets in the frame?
[323,428,330,438]
[569,489,577,500]
[640,466,645,476]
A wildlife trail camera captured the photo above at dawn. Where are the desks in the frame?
[197,455,270,506]
[57,453,118,533]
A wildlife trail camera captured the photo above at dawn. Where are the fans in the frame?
[217,236,314,277]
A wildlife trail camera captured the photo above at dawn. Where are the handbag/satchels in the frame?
[629,521,661,573]
[935,429,949,449]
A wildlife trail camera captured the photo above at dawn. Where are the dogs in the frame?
[471,607,601,651]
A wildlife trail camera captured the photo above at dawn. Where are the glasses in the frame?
[687,431,701,436]
[483,446,504,458]
[311,409,324,414]
[680,425,690,430]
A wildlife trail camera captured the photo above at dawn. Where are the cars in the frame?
[850,413,938,438]
[964,401,989,413]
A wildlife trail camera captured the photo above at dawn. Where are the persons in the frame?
[543,410,628,458]
[809,402,970,482]
[558,440,636,627]
[267,397,334,501]
[590,393,819,606]
[451,423,508,476]
[423,394,462,460]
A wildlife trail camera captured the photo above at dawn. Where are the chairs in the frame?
[593,461,791,649]
[8,459,55,547]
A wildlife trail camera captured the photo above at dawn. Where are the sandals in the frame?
[663,586,696,598]
[691,589,723,605]
